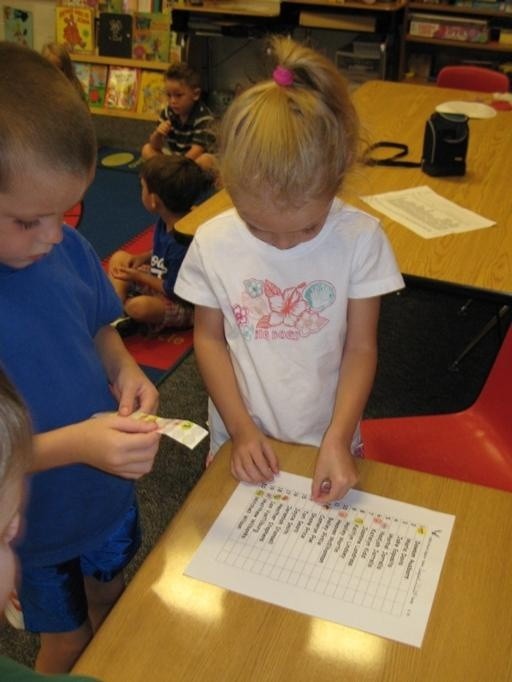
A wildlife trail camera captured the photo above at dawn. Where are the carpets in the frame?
[59,147,220,393]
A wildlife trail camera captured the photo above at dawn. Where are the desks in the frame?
[68,431,511,681]
[169,77,511,415]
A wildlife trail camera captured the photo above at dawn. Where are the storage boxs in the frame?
[331,36,392,86]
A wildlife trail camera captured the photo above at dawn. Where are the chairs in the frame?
[361,316,511,493]
[436,63,509,93]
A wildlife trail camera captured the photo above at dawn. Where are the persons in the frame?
[0,40,160,675]
[173,36,405,504]
[42,42,85,102]
[141,61,216,175]
[0,365,101,682]
[109,154,207,335]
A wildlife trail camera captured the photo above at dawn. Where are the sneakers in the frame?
[109,317,134,337]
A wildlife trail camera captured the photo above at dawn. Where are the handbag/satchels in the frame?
[420,112,469,177]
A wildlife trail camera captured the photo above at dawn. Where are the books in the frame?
[56,0,185,115]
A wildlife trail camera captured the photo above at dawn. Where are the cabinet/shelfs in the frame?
[399,0,512,94]
[53,1,183,129]
[168,2,406,95]
